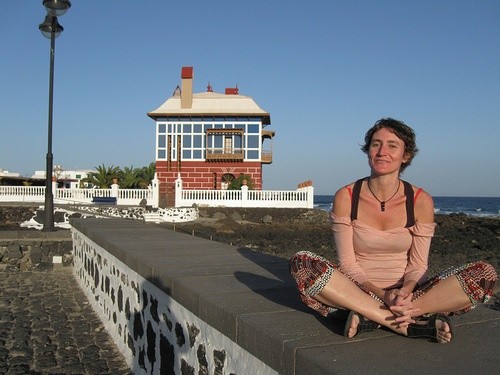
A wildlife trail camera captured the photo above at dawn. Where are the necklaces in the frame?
[367,176,401,212]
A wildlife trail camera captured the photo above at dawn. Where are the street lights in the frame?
[37,0,72,234]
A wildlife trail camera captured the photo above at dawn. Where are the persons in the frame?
[291,118,497,344]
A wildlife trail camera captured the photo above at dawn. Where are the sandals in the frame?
[407,313,453,343]
[344,311,379,338]
